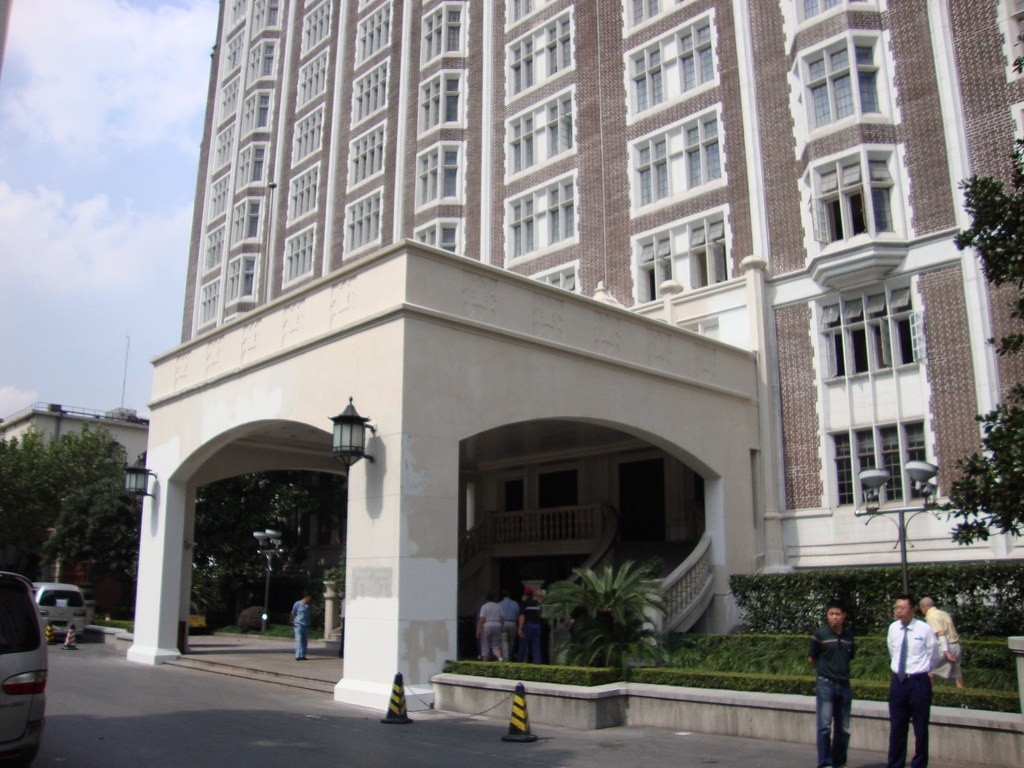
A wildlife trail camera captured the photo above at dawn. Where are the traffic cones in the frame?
[381,672,413,724]
[64,624,77,650]
[502,683,537,742]
[45,622,56,645]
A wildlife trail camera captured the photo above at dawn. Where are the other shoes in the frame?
[296,657,308,660]
[839,765,848,768]
[477,655,482,660]
[498,657,504,662]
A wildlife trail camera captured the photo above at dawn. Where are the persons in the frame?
[807,601,856,768]
[476,587,542,665]
[919,597,968,689]
[291,591,312,662]
[886,594,938,768]
[338,599,345,659]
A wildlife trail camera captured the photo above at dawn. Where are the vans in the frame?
[30,583,86,635]
[0,571,48,757]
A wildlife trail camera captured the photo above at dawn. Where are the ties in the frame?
[897,628,909,682]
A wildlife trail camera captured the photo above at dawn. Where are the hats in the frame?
[523,587,533,596]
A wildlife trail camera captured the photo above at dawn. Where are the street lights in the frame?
[855,460,939,594]
[253,530,282,632]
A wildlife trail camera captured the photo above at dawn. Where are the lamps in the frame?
[126,454,156,504]
[327,396,374,475]
